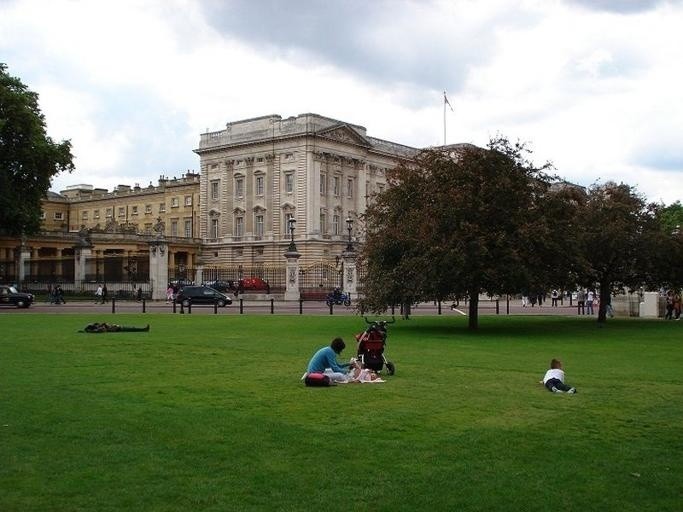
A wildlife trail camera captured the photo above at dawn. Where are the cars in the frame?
[168,277,272,290]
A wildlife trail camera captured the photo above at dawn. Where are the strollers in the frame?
[350,315,398,379]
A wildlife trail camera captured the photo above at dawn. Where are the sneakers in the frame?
[566,387,575,394]
[552,386,562,393]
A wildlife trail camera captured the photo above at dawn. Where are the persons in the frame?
[164,285,174,301]
[665,289,683,321]
[102,283,109,305]
[540,358,576,395]
[94,284,102,304]
[50,285,66,304]
[576,287,586,315]
[78,322,151,334]
[586,289,596,315]
[304,337,355,386]
[521,297,529,309]
[450,301,457,311]
[550,288,559,308]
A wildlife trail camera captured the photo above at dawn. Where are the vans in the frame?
[174,286,234,308]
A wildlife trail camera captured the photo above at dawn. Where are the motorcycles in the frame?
[326,291,352,307]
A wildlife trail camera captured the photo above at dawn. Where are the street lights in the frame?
[284,214,299,252]
[343,214,357,252]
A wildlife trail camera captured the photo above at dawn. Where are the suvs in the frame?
[0,285,36,309]
